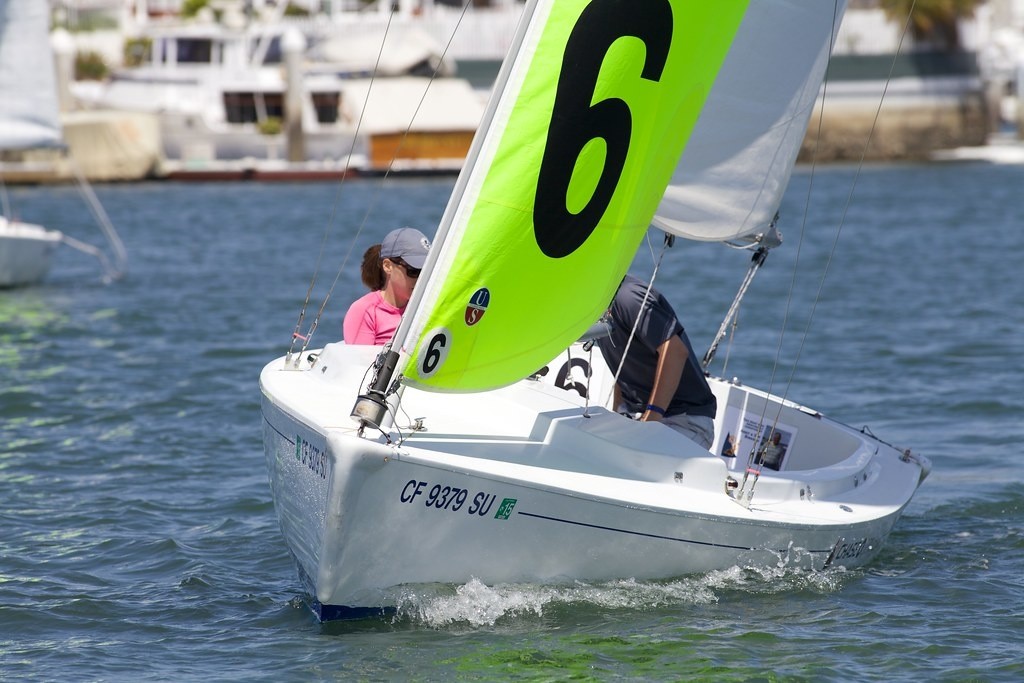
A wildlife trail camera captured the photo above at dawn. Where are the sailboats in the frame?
[255,1,931,616]
[0,0,123,288]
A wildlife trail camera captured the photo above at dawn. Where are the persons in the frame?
[760,433,785,471]
[595,274,716,451]
[722,436,737,457]
[342,227,430,345]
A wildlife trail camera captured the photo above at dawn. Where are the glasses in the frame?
[390,260,421,278]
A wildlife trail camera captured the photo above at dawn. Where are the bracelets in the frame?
[645,405,665,416]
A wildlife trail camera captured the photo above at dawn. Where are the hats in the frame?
[381,225,431,268]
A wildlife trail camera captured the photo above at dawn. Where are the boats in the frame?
[0,15,484,182]
